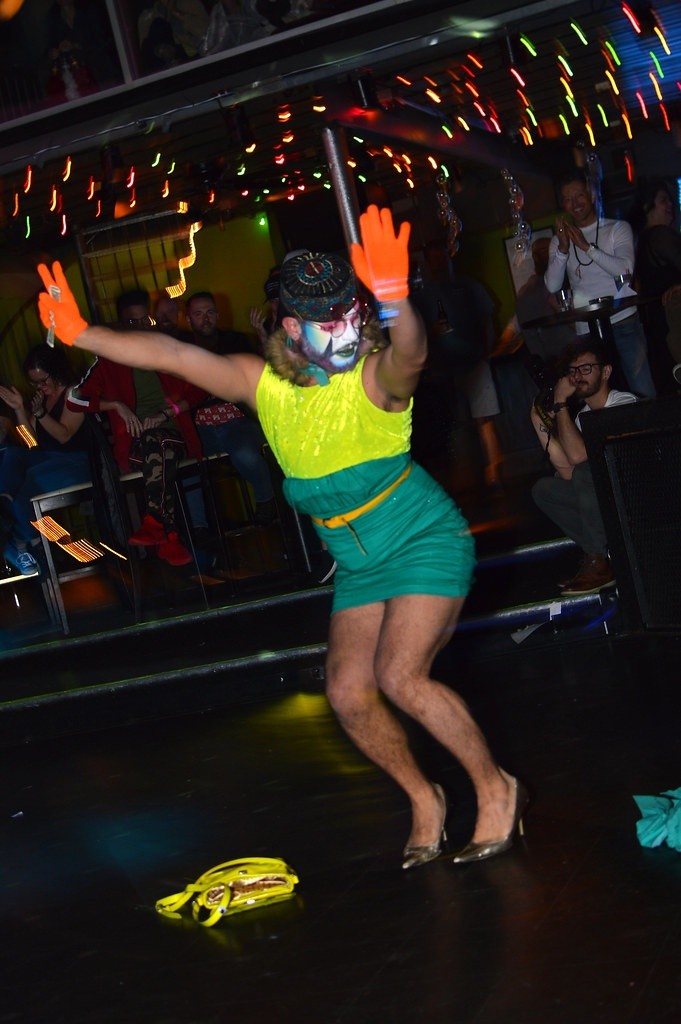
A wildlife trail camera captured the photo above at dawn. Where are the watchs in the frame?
[553,401,569,413]
[586,242,596,257]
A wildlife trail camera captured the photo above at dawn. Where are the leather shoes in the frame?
[557,546,618,595]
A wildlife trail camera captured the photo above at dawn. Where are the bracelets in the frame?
[32,408,48,419]
[160,408,170,424]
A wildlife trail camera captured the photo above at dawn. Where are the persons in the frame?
[530,337,643,595]
[544,172,658,403]
[67,283,196,570]
[35,202,536,872]
[406,244,506,488]
[0,354,87,577]
[174,288,303,575]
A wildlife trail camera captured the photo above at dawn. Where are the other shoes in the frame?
[189,527,224,545]
[314,549,338,583]
[481,479,505,497]
[255,498,275,528]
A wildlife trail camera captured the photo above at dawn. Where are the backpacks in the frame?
[154,857,300,927]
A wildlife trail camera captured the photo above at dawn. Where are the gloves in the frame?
[349,205,412,303]
[36,260,89,347]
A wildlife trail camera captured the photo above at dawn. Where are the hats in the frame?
[278,251,359,323]
[263,274,281,306]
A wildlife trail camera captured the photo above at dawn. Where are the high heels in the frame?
[452,768,532,861]
[400,783,448,870]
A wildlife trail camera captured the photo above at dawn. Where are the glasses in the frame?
[124,316,151,327]
[567,362,604,377]
[302,294,374,337]
[29,374,51,387]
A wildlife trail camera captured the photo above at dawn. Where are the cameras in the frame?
[529,368,578,412]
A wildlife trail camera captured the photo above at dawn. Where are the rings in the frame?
[129,421,134,424]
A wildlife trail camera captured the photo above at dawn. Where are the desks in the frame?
[519,291,654,404]
[27,437,278,638]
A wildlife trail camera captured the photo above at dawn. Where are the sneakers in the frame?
[127,514,170,547]
[157,532,194,566]
[2,542,39,575]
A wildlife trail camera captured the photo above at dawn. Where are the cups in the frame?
[557,289,573,311]
[613,273,631,291]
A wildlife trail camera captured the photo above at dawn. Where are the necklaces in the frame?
[570,215,601,280]
